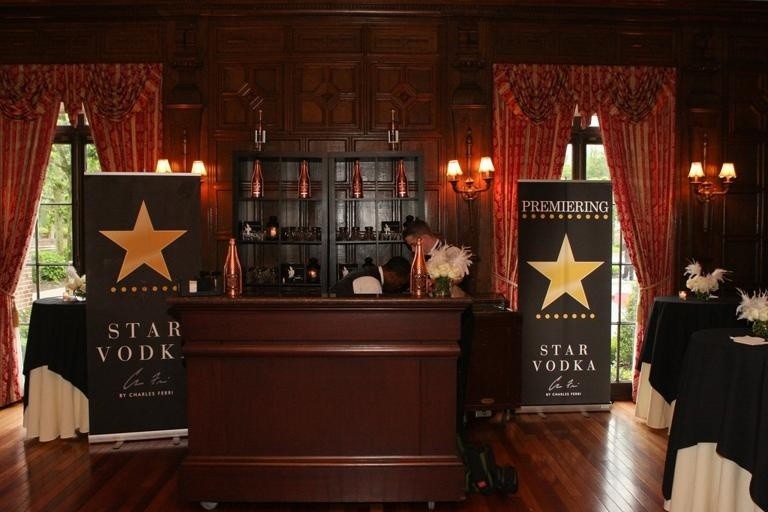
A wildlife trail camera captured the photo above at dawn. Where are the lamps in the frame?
[688,161,737,202]
[154,158,208,176]
[446,156,495,201]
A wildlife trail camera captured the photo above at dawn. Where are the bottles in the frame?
[296,160,311,198]
[386,108,401,150]
[409,237,428,298]
[265,215,280,242]
[253,109,267,152]
[394,159,409,198]
[221,237,244,299]
[350,159,363,198]
[250,159,264,198]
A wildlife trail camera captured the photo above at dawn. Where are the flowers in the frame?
[425,239,474,281]
[735,288,768,322]
[683,258,734,295]
[67,271,87,298]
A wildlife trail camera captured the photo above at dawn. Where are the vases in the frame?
[752,320,768,341]
[76,295,87,302]
[696,289,709,302]
[432,277,452,298]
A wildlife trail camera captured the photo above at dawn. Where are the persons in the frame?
[402,218,475,445]
[327,255,412,297]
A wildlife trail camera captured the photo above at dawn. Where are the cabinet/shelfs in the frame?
[233,151,328,292]
[328,151,425,290]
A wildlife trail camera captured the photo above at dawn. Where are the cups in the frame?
[280,225,374,240]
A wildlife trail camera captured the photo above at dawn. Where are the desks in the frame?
[22,296,90,442]
[634,295,755,436]
[662,326,768,512]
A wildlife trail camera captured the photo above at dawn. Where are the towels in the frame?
[729,335,765,343]
